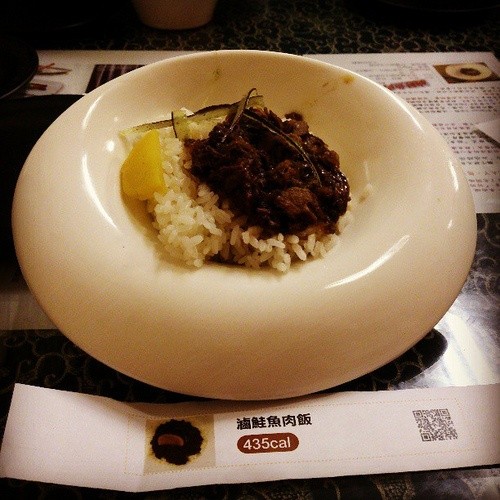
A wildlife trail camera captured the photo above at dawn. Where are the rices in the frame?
[142,126,373,272]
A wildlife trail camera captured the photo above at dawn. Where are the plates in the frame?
[12,50,477,402]
